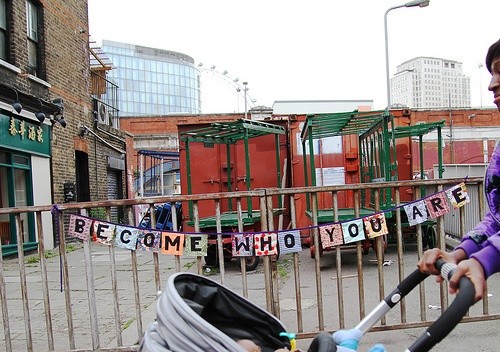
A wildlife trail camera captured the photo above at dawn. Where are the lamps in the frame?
[46,102,68,127]
[1,83,23,114]
[23,94,45,123]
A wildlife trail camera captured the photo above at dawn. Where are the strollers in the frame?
[138,255,477,352]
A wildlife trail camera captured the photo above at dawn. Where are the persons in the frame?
[418,37,500,307]
[232,338,290,352]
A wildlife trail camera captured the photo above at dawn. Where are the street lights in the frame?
[384,0,430,110]
